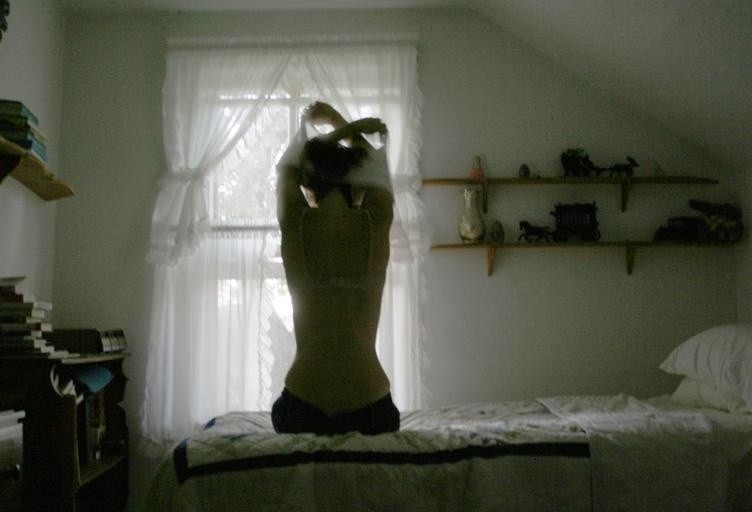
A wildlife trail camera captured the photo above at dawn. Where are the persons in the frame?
[270,99,402,435]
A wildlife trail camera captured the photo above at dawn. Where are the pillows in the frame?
[669,376,749,416]
[660,323,752,411]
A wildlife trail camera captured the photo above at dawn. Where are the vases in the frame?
[460,187,485,244]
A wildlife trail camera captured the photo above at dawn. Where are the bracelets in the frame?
[328,109,341,125]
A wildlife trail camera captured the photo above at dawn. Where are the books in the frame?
[0,276,81,360]
[1,99,50,168]
[52,367,116,473]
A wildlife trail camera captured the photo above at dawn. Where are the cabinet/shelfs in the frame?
[422,178,742,277]
[0,353,129,512]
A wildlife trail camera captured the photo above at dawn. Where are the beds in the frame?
[150,394,752,509]
